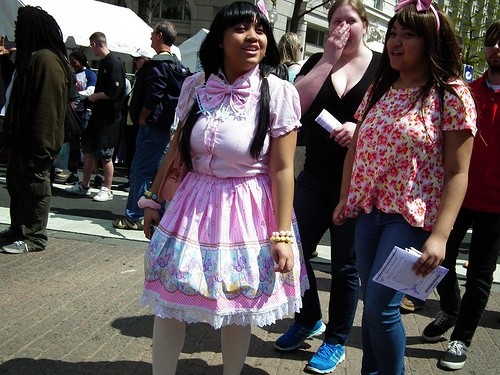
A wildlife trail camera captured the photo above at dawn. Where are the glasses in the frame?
[484,40,500,47]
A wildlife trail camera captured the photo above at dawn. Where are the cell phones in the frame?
[149,220,153,239]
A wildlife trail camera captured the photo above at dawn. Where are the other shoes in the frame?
[400,298,418,311]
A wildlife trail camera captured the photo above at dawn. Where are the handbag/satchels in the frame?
[126,110,133,125]
[63,98,85,143]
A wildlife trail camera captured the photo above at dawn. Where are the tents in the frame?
[0,0,181,63]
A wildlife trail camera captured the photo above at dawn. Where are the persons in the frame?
[275,33,309,84]
[423,22,500,375]
[0,6,191,254]
[275,0,391,375]
[138,0,310,375]
[332,0,478,374]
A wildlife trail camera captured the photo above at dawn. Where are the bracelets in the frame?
[138,196,162,211]
[270,236,293,244]
[272,231,295,238]
[144,190,160,204]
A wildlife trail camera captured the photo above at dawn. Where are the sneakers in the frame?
[306,342,345,373]
[422,311,456,340]
[440,340,468,369]
[63,174,79,184]
[94,175,103,187]
[0,229,25,241]
[112,216,145,230]
[274,318,326,350]
[2,241,45,253]
[93,186,113,202]
[65,181,91,195]
